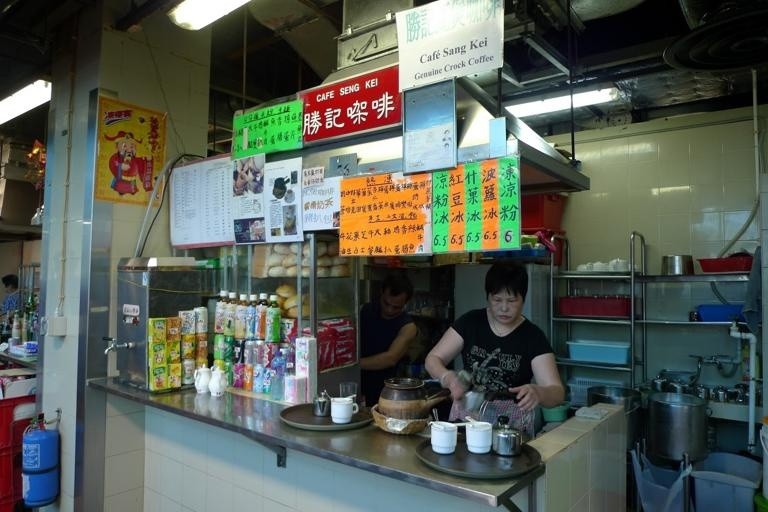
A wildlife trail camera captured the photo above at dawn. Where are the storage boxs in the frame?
[521,193,568,231]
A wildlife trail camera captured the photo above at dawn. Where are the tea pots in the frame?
[193,363,211,394]
[311,391,330,417]
[493,414,523,457]
[651,376,759,406]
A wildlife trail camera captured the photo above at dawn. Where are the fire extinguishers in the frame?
[21,413,61,508]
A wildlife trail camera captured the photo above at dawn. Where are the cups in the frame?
[431,421,458,454]
[340,381,357,410]
[573,287,630,299]
[575,258,630,272]
[284,190,295,203]
[330,397,359,424]
[236,173,249,188]
[464,421,493,455]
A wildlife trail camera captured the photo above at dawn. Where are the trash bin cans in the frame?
[690,452,763,512]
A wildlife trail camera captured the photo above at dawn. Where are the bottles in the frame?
[194,392,281,434]
[209,289,294,397]
[1,307,39,345]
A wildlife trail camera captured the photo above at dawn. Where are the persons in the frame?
[424,261,565,422]
[105,131,154,195]
[359,277,419,406]
[0,274,21,342]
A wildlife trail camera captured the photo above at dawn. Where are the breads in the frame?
[275,284,310,318]
[269,243,350,277]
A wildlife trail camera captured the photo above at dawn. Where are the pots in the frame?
[273,176,289,195]
[377,376,451,420]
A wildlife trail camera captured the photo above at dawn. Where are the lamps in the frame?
[0,72,53,125]
[501,82,621,118]
[166,0,252,32]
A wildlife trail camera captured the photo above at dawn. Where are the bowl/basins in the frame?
[662,255,695,277]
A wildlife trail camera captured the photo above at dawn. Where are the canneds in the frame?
[182,333,209,384]
[213,334,295,394]
[194,307,209,333]
[224,392,283,436]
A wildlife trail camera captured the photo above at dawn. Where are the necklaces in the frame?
[492,316,523,335]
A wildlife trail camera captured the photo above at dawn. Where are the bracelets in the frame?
[440,370,452,388]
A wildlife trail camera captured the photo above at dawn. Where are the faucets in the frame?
[701,355,724,370]
[101,336,136,355]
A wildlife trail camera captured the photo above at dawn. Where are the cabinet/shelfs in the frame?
[550,231,763,407]
[173,234,361,399]
[363,264,455,370]
